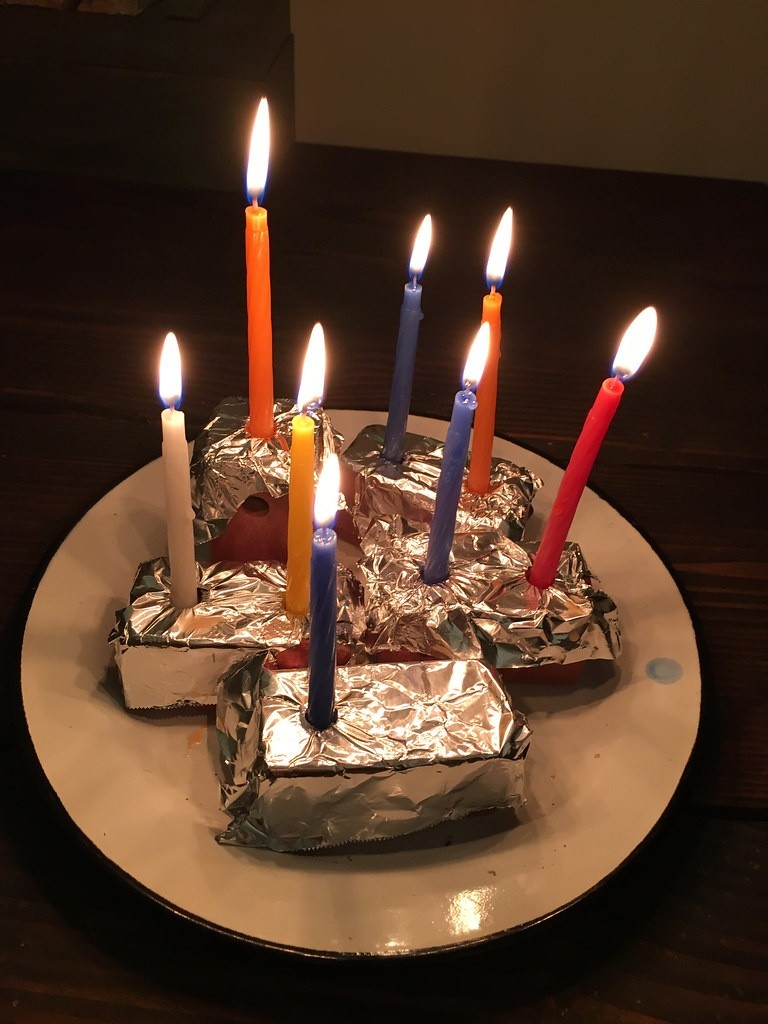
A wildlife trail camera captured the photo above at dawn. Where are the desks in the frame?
[0,117,768,1024]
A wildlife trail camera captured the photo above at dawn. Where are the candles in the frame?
[244,205,275,437]
[306,527,337,728]
[468,292,502,495]
[530,377,625,587]
[283,414,316,614]
[383,284,424,463]
[162,407,199,607]
[424,391,477,584]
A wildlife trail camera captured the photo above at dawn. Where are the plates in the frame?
[20,408,700,961]
